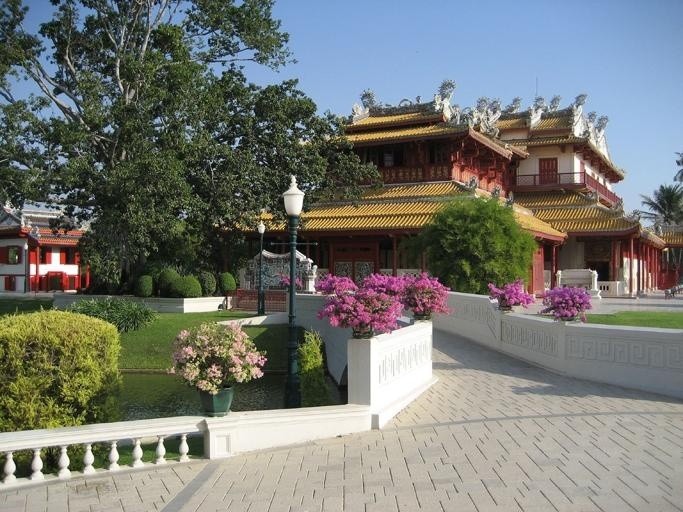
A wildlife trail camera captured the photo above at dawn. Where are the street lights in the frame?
[23,244,28,294]
[257,220,267,316]
[282,176,305,408]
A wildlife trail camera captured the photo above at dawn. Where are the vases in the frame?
[352,330,373,339]
[201,384,234,417]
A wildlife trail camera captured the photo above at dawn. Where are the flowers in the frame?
[281,271,452,334]
[166,321,267,396]
[487,279,593,323]
